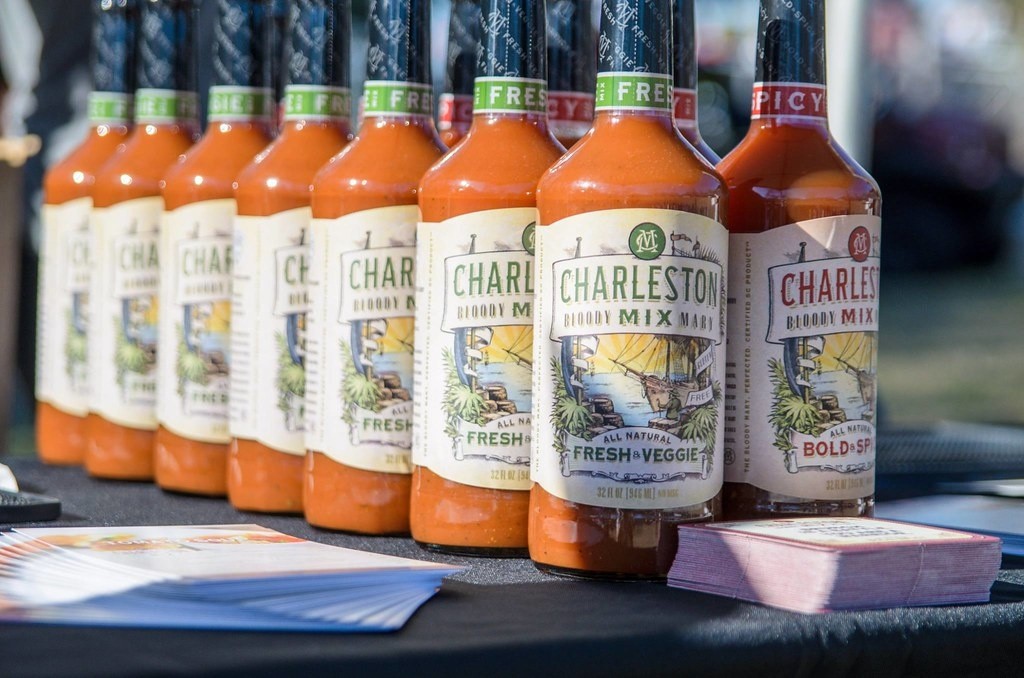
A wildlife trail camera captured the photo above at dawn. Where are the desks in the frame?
[1,399,1023,678]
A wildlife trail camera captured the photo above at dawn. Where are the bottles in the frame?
[152,0,282,497]
[544,0,596,151]
[224,0,354,513]
[531,0,724,584]
[32,0,141,464]
[669,0,722,165]
[84,0,202,479]
[308,0,450,536]
[720,2,879,521]
[407,0,570,549]
[437,0,492,149]
